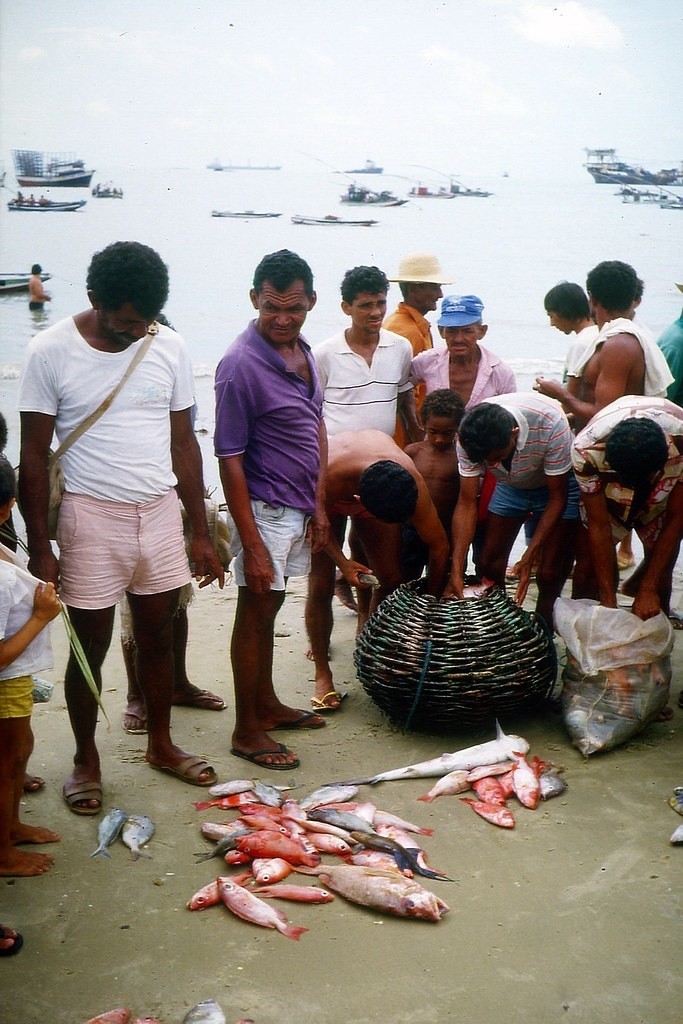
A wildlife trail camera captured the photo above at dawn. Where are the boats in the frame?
[340,190,406,209]
[13,151,96,188]
[409,184,456,199]
[0,272,51,292]
[449,183,494,198]
[9,196,87,212]
[93,181,123,200]
[212,209,281,219]
[293,212,378,225]
[584,149,682,186]
[617,187,683,210]
[345,160,383,173]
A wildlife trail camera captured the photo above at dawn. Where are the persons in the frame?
[120,316,228,734]
[18,240,224,813]
[28,264,50,310]
[17,191,47,206]
[452,390,584,637]
[0,412,62,956]
[304,428,449,713]
[305,264,413,664]
[213,248,331,771]
[570,393,683,723]
[401,387,467,587]
[379,253,683,629]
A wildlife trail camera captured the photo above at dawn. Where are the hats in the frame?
[437,295,484,326]
[387,254,456,285]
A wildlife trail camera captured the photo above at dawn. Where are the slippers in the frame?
[265,709,326,730]
[123,711,147,733]
[63,781,103,814]
[669,616,683,630]
[310,692,341,710]
[230,743,300,769]
[617,552,634,569]
[150,756,217,786]
[192,690,227,710]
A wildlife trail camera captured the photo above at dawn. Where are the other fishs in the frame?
[334,567,380,614]
[667,786,683,843]
[181,776,458,942]
[82,997,259,1024]
[320,716,569,830]
[88,806,156,862]
[177,483,233,580]
[562,620,670,754]
[439,575,496,601]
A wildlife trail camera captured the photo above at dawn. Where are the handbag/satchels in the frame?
[14,448,65,539]
